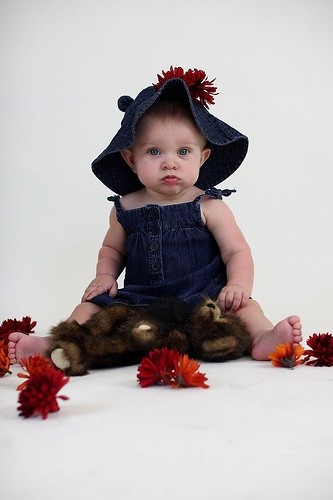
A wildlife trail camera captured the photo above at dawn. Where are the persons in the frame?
[7,66,304,365]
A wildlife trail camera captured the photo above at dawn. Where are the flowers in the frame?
[267,332,333,369]
[134,348,210,390]
[0,316,38,376]
[152,64,219,110]
[15,354,70,422]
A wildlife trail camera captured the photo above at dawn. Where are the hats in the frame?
[91,66,249,197]
